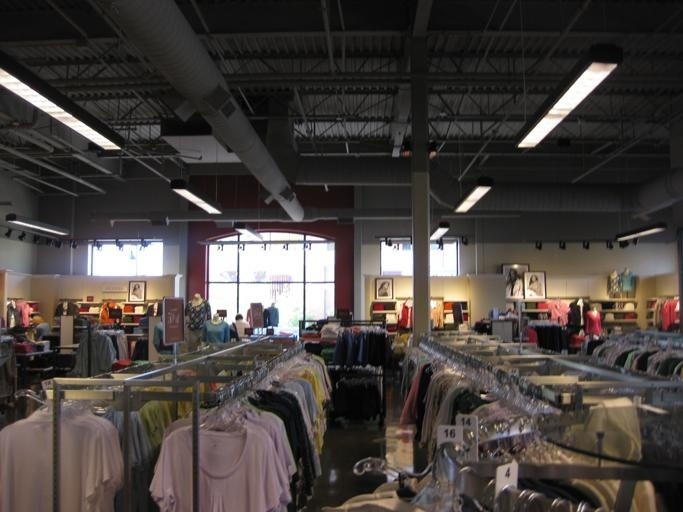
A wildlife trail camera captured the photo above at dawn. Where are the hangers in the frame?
[416,333,562,425]
[14,380,110,421]
[349,415,606,512]
[197,342,314,436]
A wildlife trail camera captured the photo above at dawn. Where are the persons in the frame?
[198,312,231,345]
[31,314,51,341]
[505,267,524,298]
[377,280,389,296]
[618,266,635,293]
[129,283,142,300]
[184,293,211,350]
[569,297,590,336]
[582,306,601,336]
[227,313,251,341]
[527,274,542,297]
[266,303,280,327]
[605,269,622,295]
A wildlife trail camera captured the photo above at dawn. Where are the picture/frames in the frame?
[375,278,394,300]
[129,281,146,302]
[501,262,547,299]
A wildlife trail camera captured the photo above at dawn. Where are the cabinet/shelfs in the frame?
[411,336,683,512]
[521,297,657,329]
[23,337,307,512]
[7,301,163,379]
[372,297,468,331]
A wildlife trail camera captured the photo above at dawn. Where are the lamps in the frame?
[0,56,124,150]
[5,213,150,249]
[167,178,312,251]
[512,46,621,152]
[384,180,494,248]
[533,222,665,250]
